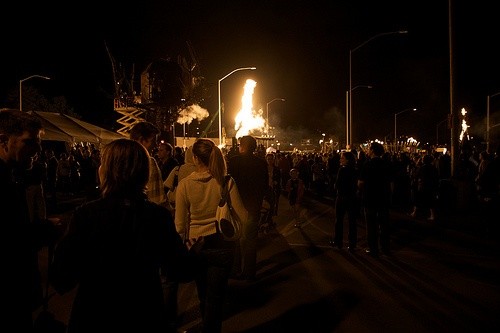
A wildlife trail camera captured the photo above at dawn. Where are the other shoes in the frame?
[346,245,358,251]
[329,240,337,247]
[379,247,392,256]
[294,224,301,228]
[360,248,378,255]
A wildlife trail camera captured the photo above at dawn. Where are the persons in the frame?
[27,122,500,333]
[0,108,63,333]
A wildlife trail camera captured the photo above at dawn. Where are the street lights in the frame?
[218,67,255,148]
[19,75,50,111]
[393,107,418,149]
[345,83,373,146]
[349,29,409,146]
[266,98,285,148]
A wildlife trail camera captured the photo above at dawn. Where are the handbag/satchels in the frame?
[215,173,242,241]
[167,165,180,204]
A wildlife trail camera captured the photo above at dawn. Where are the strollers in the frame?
[260,180,280,234]
